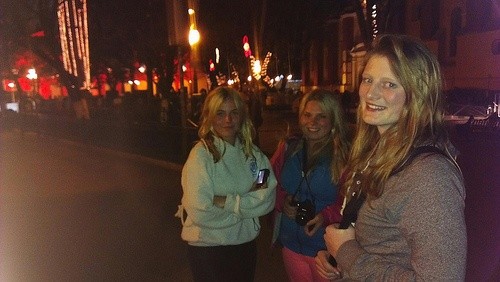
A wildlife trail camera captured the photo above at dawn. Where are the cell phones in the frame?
[256,169,269,185]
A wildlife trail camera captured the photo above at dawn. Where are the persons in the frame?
[332,89,360,122]
[160,87,207,128]
[313,33,468,282]
[180,86,277,282]
[268,87,350,282]
[270,85,304,109]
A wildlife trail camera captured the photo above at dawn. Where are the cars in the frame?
[187,93,205,122]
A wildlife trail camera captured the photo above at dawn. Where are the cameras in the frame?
[290,200,315,226]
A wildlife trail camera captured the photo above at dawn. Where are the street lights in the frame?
[188,25,201,93]
